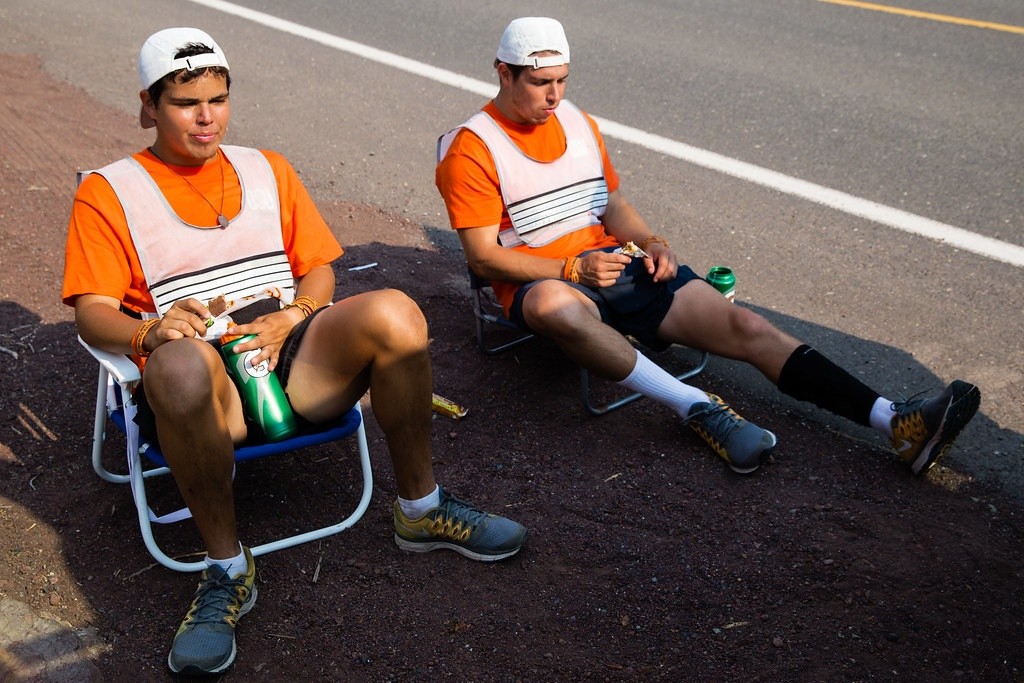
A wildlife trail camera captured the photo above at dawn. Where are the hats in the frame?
[494,17,571,68]
[141,28,230,129]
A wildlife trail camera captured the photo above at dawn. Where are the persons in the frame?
[61,28,528,677]
[435,15,980,475]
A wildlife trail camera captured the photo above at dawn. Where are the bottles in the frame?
[705,266,736,304]
[219,321,295,441]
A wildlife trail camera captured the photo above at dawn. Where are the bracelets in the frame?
[564,257,580,282]
[286,295,320,318]
[130,319,160,357]
[640,236,670,253]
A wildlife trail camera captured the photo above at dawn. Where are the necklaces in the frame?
[148,146,229,229]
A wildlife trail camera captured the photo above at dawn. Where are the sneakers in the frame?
[887,380,981,477]
[677,392,777,473]
[167,546,258,678]
[394,484,528,561]
